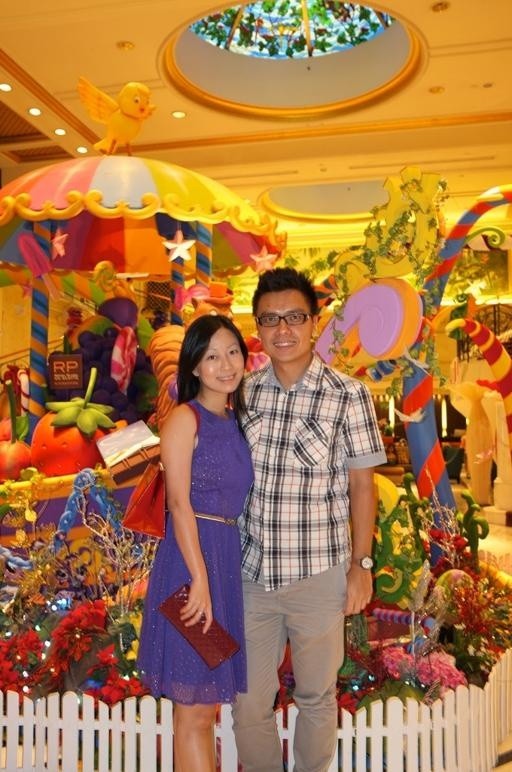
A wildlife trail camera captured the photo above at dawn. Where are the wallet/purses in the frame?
[159,585,240,671]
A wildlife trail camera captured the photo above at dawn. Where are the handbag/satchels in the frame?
[121,402,201,538]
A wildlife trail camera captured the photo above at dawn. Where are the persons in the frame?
[134,314,250,772]
[236,267,387,771]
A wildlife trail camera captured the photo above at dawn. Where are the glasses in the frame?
[257,312,313,327]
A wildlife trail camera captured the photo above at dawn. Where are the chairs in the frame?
[442,447,464,483]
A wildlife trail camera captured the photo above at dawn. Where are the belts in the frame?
[194,512,238,525]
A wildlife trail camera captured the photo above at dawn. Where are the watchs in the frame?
[353,556,376,573]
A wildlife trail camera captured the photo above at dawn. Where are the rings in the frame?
[197,610,203,616]
